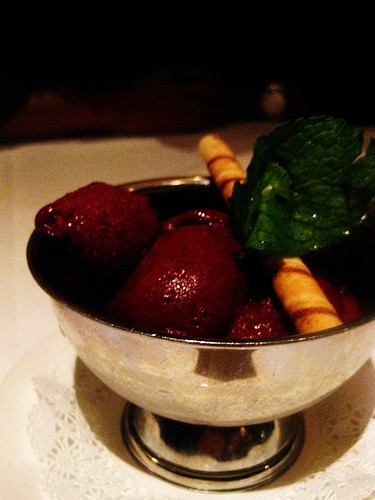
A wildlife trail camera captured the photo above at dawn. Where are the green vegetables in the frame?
[227,115,375,260]
